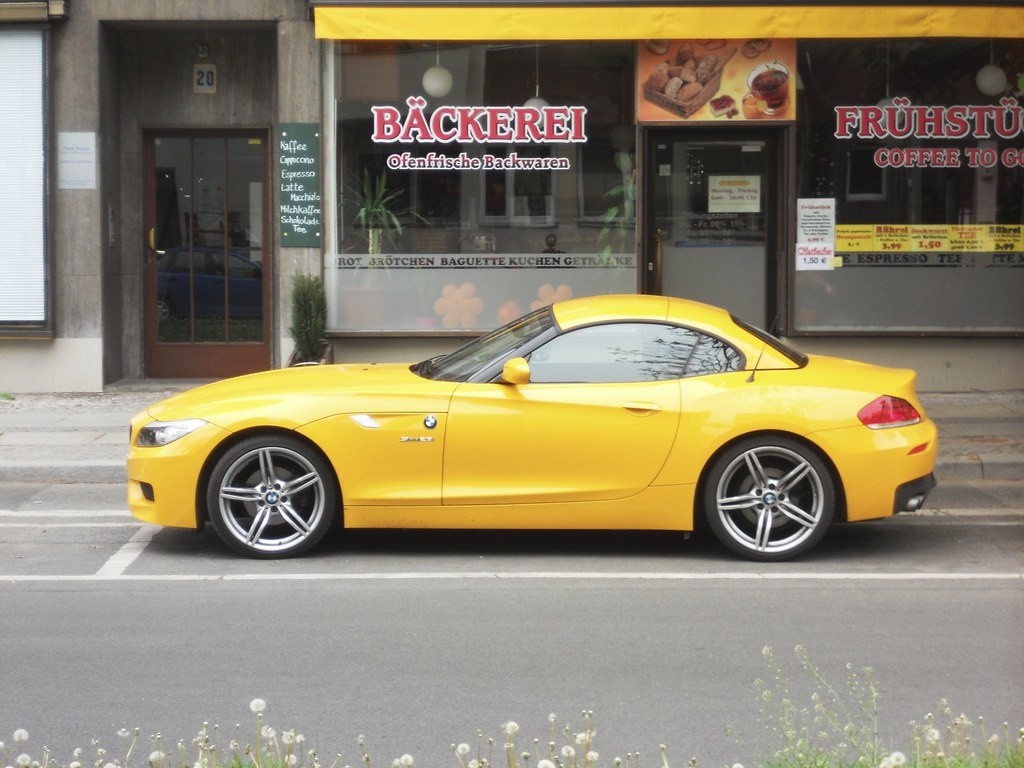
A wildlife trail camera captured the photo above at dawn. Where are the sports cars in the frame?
[125,292,940,562]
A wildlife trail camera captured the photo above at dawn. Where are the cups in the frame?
[746,62,790,115]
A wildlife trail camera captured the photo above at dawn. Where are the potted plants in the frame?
[284,269,334,368]
[338,166,412,255]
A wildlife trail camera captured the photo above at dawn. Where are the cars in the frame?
[149,244,265,322]
